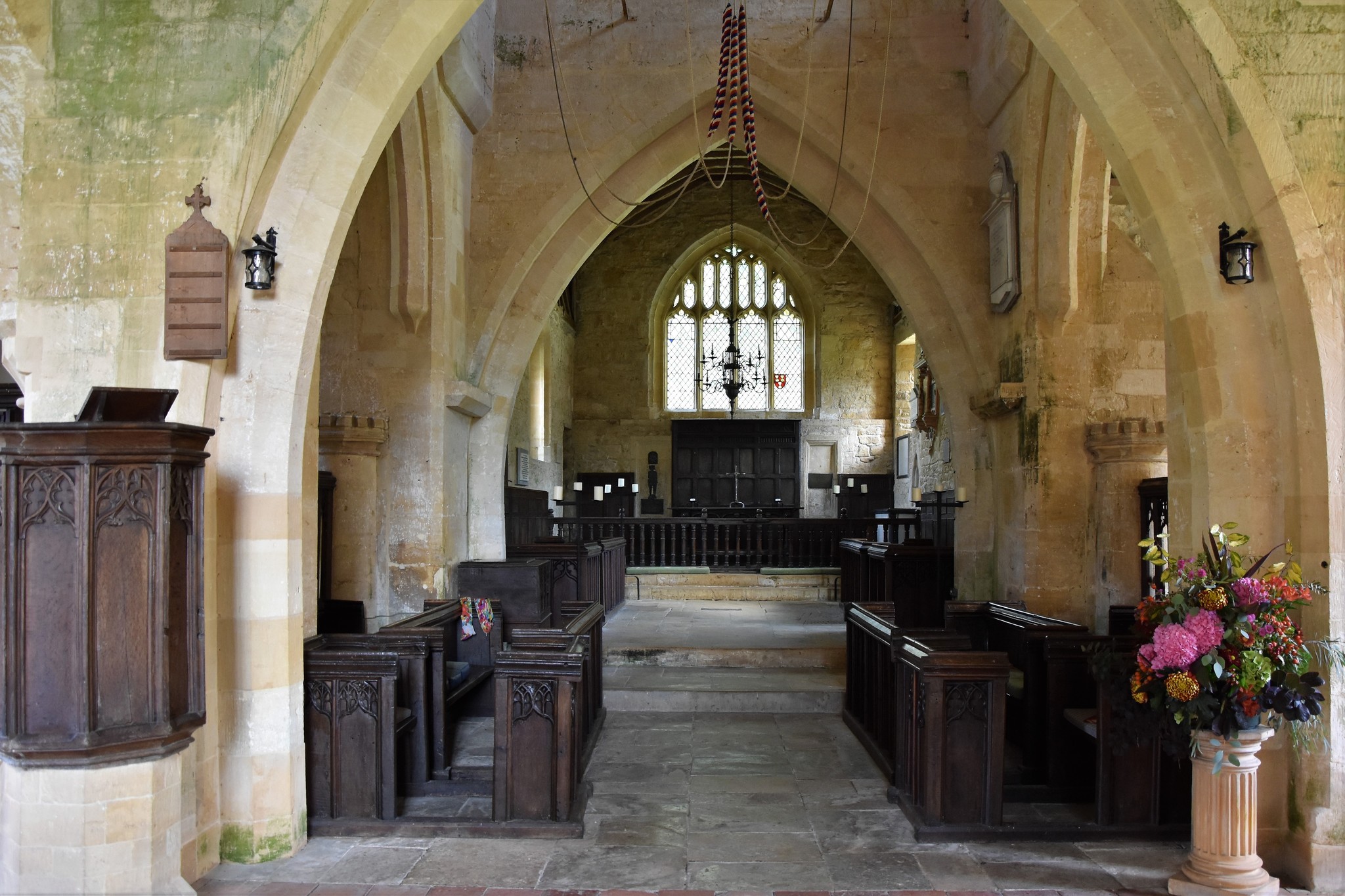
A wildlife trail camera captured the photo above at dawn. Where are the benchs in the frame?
[1044,634,1162,826]
[303,633,439,819]
[377,598,503,772]
[944,600,1089,784]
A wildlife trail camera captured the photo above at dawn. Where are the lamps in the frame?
[1217,220,1258,285]
[240,226,277,290]
[541,0,895,421]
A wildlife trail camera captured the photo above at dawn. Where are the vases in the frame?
[1243,712,1262,730]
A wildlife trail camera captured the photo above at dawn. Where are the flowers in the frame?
[1129,521,1345,775]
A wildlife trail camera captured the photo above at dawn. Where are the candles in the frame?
[594,486,603,501]
[604,484,611,493]
[861,484,868,493]
[833,485,840,493]
[553,486,563,500]
[775,499,781,501]
[632,484,638,492]
[690,498,696,501]
[956,487,967,501]
[912,487,922,501]
[935,484,944,492]
[618,478,625,487]
[573,482,582,491]
[848,478,854,487]
